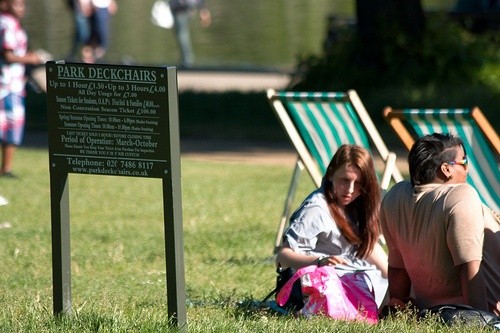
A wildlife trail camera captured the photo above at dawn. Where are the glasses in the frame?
[446,156,468,166]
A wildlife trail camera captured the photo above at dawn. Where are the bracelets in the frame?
[317,256,325,267]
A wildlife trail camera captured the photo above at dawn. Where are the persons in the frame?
[169,0,199,66]
[274,145,388,316]
[379,133,500,319]
[0,0,53,179]
[64,0,117,64]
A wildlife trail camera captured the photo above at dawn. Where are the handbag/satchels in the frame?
[277,266,378,325]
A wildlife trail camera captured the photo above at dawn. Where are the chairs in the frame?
[264,87,406,256]
[385,105,500,216]
